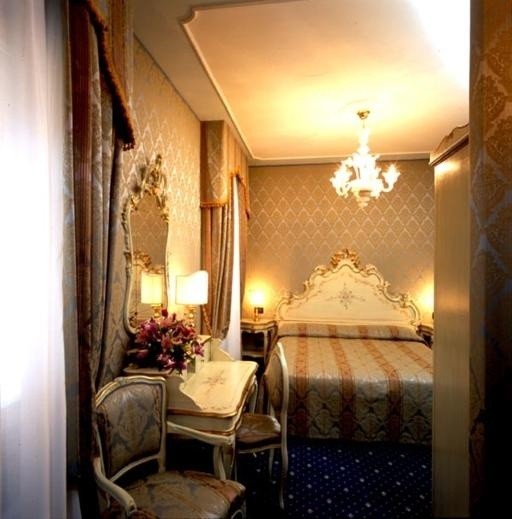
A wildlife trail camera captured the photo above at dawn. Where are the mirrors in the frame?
[120,152,173,335]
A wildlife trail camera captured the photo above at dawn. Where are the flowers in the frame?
[127,305,204,376]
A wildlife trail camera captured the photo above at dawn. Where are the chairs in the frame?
[88,342,289,518]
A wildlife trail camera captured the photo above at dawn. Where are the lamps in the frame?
[175,269,208,328]
[140,260,166,320]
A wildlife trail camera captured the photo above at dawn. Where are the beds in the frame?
[261,250,435,446]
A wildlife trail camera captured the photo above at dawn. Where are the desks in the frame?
[240,317,277,367]
[420,324,435,348]
